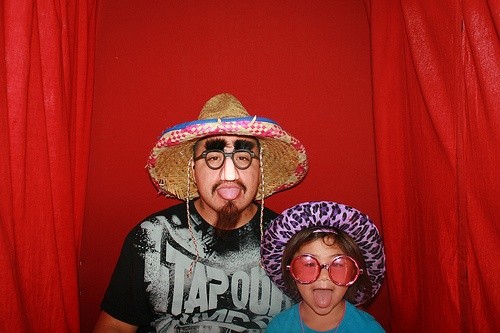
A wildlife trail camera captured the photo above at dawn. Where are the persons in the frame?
[92,93,310,333]
[260,201,386,333]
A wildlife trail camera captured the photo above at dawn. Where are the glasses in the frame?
[286,253,364,287]
[193,148,260,171]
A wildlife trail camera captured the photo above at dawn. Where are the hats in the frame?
[145,93,309,203]
[260,200,386,307]
